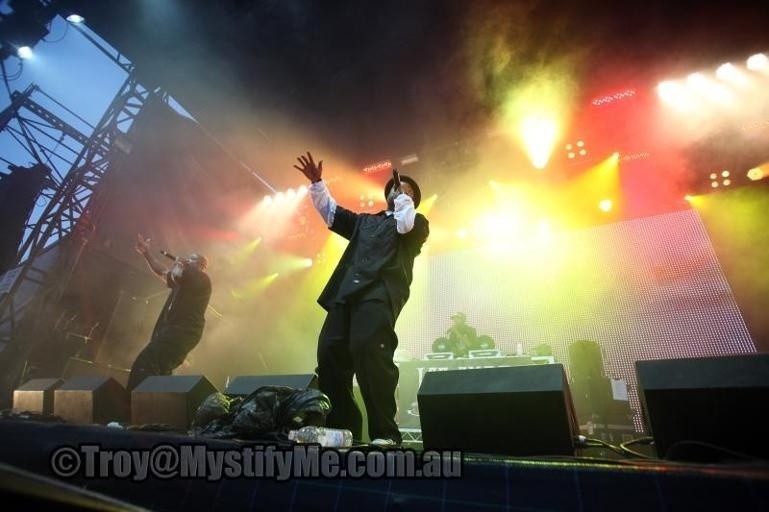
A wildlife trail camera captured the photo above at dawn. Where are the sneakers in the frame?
[368,435,401,449]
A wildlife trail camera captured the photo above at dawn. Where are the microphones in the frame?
[446,324,456,334]
[393,168,401,190]
[159,250,187,266]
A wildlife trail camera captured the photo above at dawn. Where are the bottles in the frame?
[516,341,522,356]
[288,424,354,452]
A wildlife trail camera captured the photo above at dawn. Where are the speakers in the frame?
[418,363,582,458]
[132,374,220,429]
[53,376,131,425]
[635,352,769,464]
[13,379,64,416]
[223,373,326,427]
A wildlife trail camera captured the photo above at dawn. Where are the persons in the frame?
[292,150,429,448]
[446,312,477,346]
[126,233,212,392]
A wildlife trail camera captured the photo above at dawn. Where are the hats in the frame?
[384,175,422,210]
